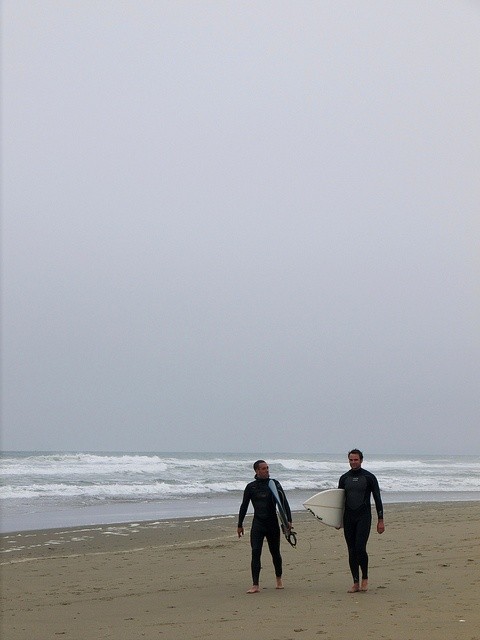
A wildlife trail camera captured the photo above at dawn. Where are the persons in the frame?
[237,459,292,593]
[334,448,385,593]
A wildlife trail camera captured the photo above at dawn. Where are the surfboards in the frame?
[302,489,345,528]
[268,480,297,549]
